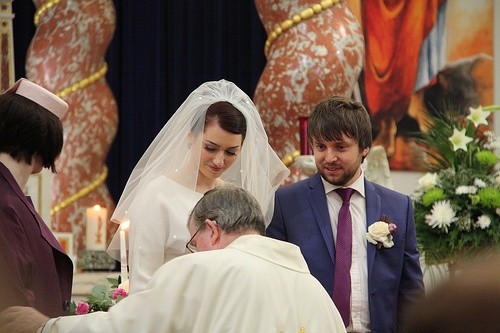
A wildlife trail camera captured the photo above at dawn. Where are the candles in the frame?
[117,215,130,283]
[85,203,108,251]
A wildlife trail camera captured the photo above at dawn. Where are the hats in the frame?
[5,76,69,119]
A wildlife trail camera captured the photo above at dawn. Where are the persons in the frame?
[266,96,425,333]
[0,187,348,333]
[103,0,269,207]
[0,77,74,319]
[108,79,292,294]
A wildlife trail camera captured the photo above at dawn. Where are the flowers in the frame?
[364,214,397,251]
[405,97,500,285]
[66,274,128,315]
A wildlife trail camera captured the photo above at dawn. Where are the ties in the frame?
[333,188,355,328]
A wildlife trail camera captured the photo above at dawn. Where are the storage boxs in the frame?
[76,248,117,271]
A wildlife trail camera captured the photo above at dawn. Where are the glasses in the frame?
[185,215,219,254]
